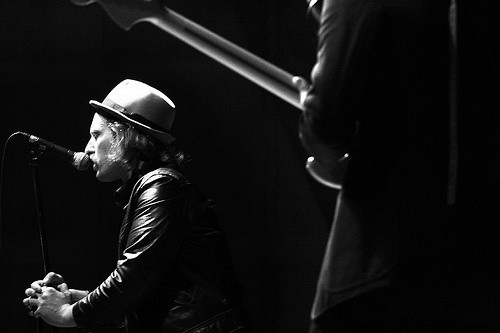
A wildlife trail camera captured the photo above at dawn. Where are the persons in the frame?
[298,0,500,333]
[21,77,252,333]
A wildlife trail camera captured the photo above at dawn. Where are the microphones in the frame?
[19,131,91,171]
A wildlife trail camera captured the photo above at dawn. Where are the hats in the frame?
[89,79,176,141]
